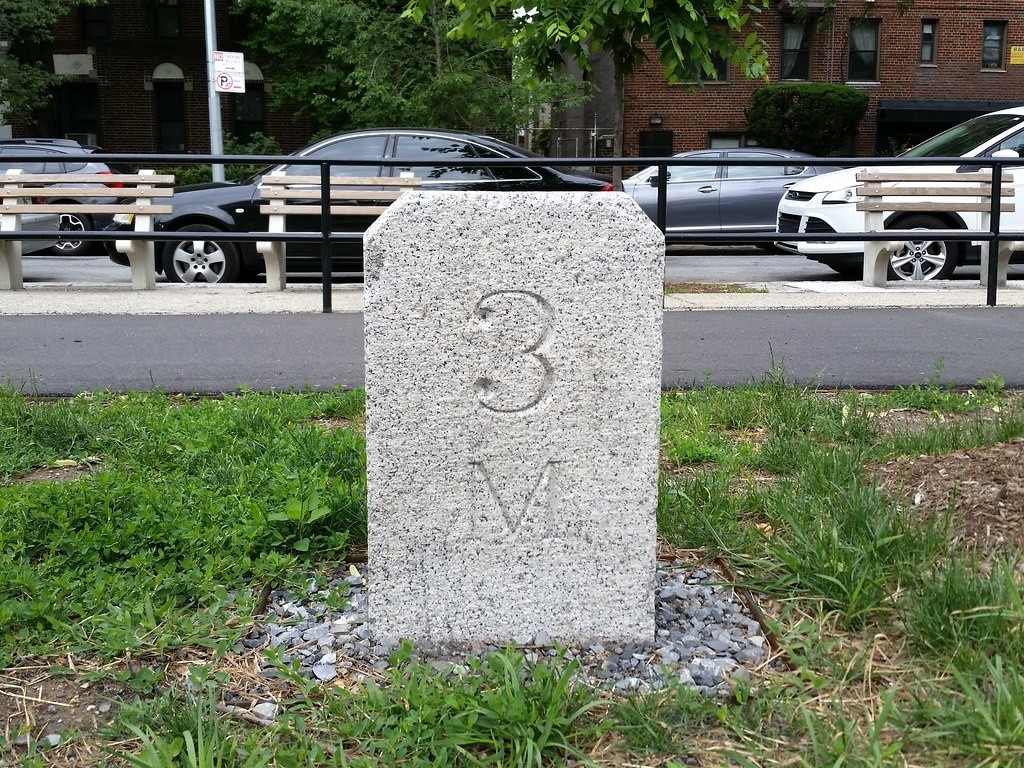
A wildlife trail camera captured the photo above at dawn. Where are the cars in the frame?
[0,138,138,256]
[102,130,625,284]
[774,107,1024,281]
[621,148,853,255]
